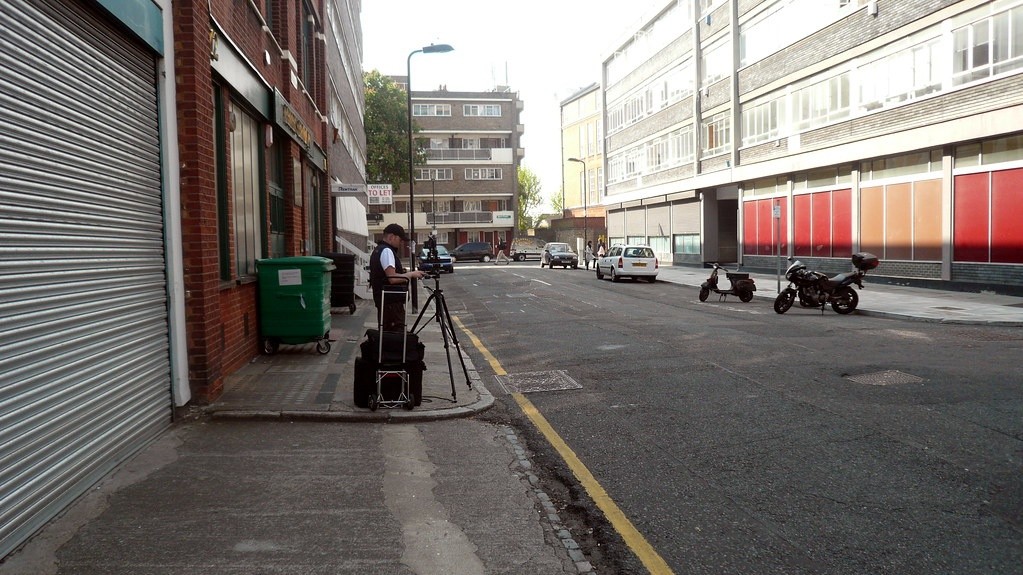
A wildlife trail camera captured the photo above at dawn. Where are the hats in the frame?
[384,224,411,242]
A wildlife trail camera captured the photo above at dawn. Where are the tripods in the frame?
[410,275,472,403]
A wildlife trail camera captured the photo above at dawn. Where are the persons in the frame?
[369,223,428,331]
[586,241,596,270]
[597,238,605,254]
[494,236,510,265]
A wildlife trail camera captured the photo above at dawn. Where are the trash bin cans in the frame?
[313,253,357,315]
[254,256,337,355]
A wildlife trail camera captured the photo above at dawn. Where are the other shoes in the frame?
[494,262,498,265]
[507,261,510,265]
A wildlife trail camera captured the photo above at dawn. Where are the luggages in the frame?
[353,286,426,412]
